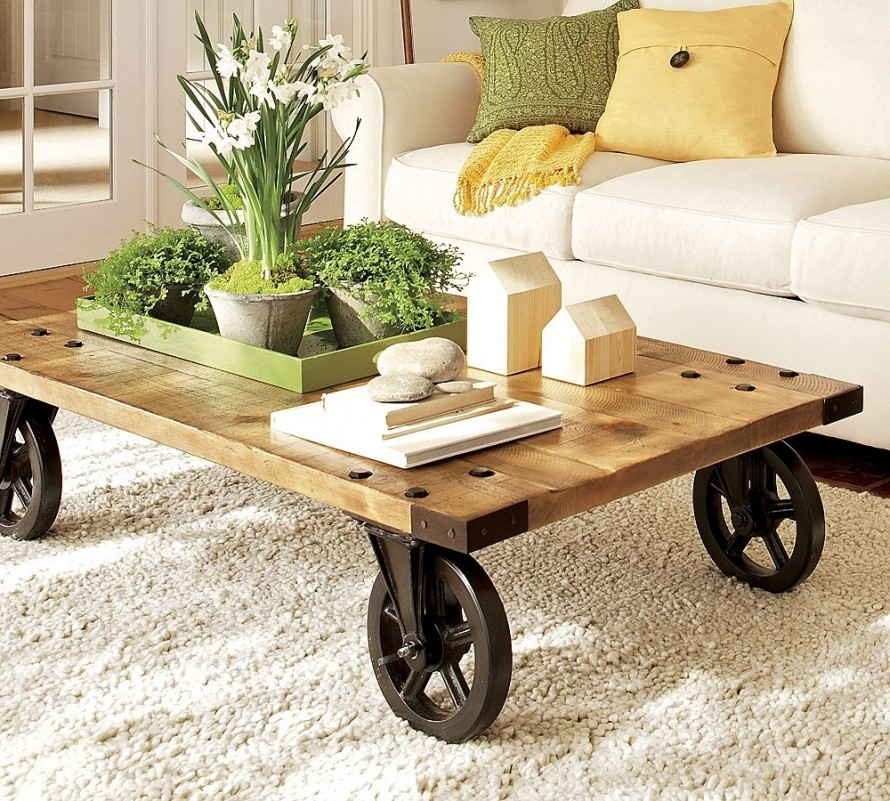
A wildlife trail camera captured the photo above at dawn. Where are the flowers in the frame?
[133,22,370,279]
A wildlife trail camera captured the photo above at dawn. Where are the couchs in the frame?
[326,0,890,452]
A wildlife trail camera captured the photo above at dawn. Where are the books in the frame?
[270,377,563,469]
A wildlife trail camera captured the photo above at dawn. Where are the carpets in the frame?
[0,409,890,801]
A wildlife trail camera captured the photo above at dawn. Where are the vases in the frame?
[205,277,310,354]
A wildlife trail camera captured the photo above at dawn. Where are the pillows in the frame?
[467,0,794,160]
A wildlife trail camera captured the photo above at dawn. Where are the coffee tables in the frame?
[0,291,862,743]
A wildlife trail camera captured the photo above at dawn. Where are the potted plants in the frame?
[78,227,199,339]
[183,188,306,262]
[294,217,472,347]
